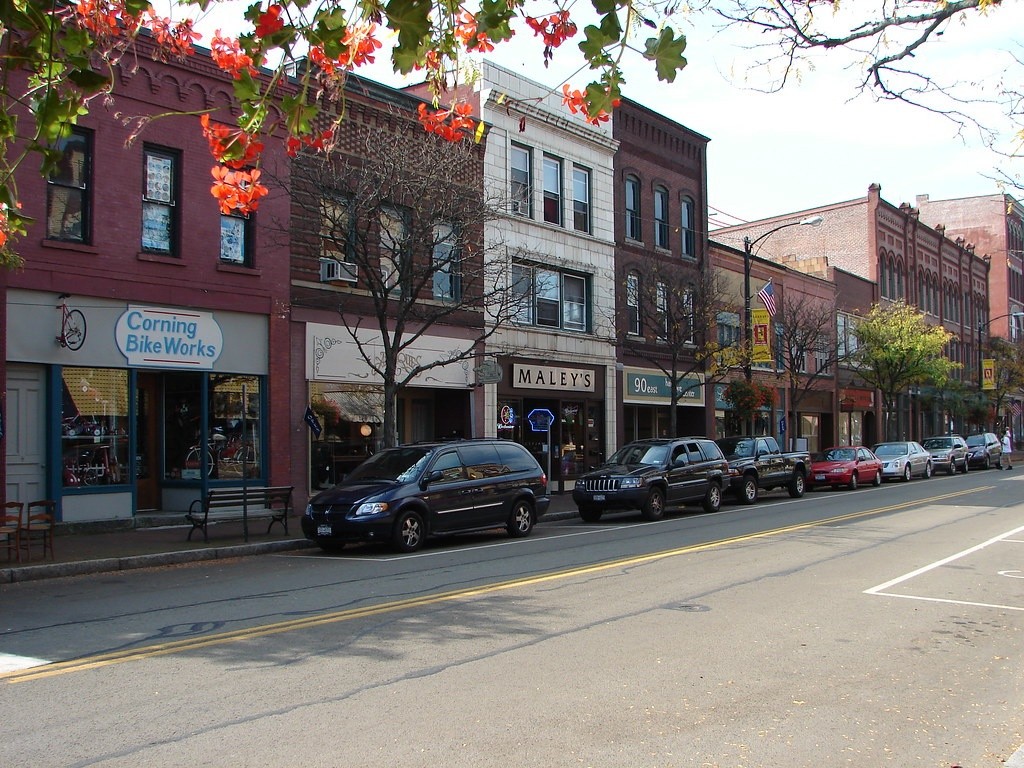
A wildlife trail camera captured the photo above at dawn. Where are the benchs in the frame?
[184,484,295,544]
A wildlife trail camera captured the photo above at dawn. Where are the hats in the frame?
[1006,427,1009,430]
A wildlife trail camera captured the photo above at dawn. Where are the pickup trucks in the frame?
[715,435,813,504]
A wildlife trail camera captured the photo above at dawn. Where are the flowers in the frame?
[720,379,782,424]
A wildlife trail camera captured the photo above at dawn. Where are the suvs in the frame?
[918,433,970,475]
[959,432,1004,471]
[300,436,550,554]
[571,436,729,523]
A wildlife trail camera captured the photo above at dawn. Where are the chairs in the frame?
[16,500,57,563]
[0,501,25,563]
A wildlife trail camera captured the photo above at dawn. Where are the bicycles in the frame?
[186,420,256,477]
[57,293,87,351]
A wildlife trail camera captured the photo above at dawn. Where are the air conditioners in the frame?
[319,260,357,282]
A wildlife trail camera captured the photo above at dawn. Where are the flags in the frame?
[779,416,786,436]
[302,405,323,441]
[1004,396,1022,419]
[750,309,772,363]
[757,281,777,317]
[983,359,995,390]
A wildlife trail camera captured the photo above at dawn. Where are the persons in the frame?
[1000,426,1014,471]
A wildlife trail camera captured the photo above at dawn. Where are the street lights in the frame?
[743,217,825,428]
[976,311,1023,433]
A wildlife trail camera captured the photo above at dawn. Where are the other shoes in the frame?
[1006,465,1012,470]
[998,466,1002,470]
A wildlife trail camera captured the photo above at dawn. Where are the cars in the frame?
[808,447,883,491]
[871,441,934,482]
